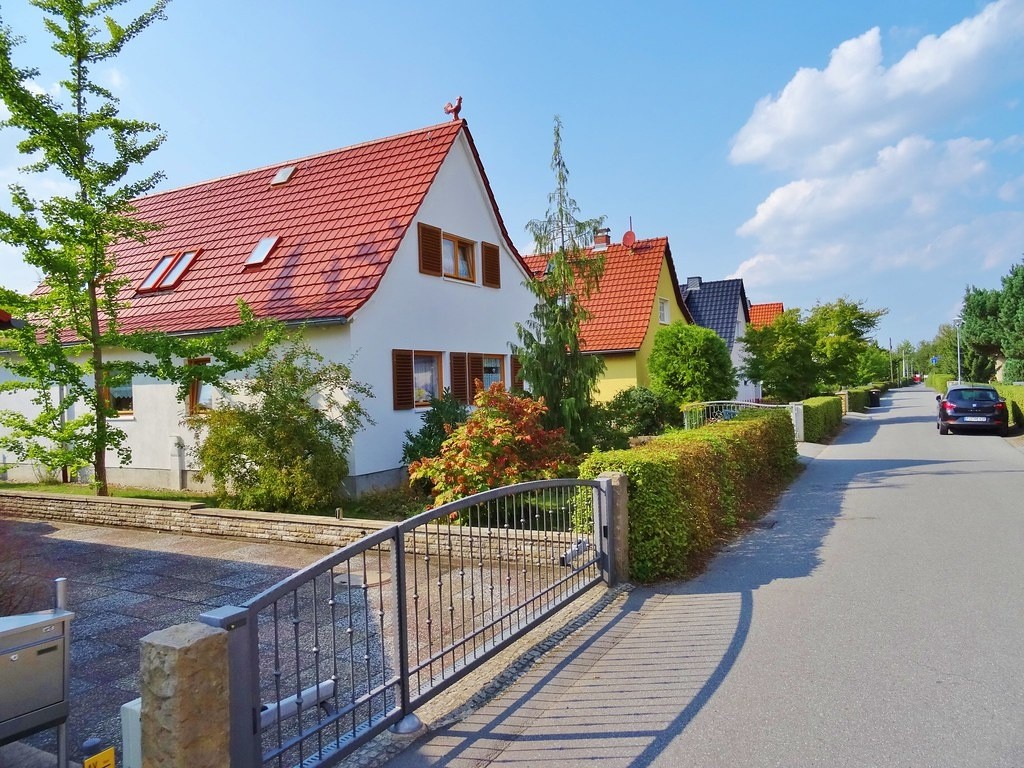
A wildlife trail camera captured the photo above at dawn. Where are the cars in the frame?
[935,385,1009,438]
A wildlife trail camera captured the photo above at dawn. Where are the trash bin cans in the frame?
[870,389,881,407]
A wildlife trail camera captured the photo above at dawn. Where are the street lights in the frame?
[953,318,963,386]
